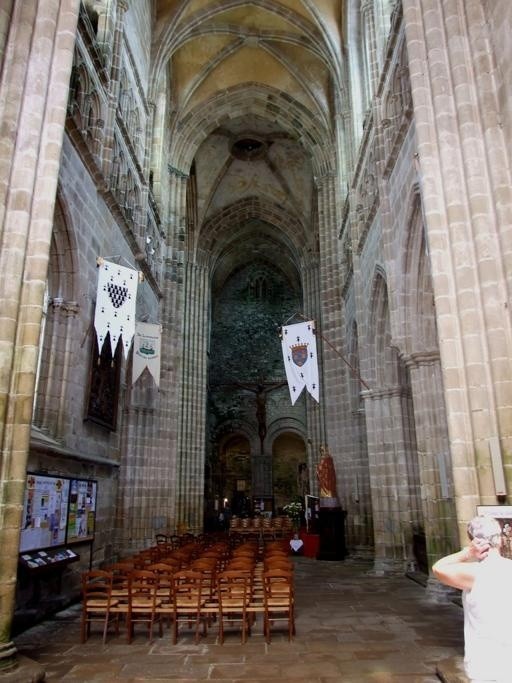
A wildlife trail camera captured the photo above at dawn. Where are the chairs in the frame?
[79,514,298,646]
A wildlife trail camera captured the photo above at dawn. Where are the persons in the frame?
[20,494,93,537]
[314,443,337,498]
[431,512,512,683]
